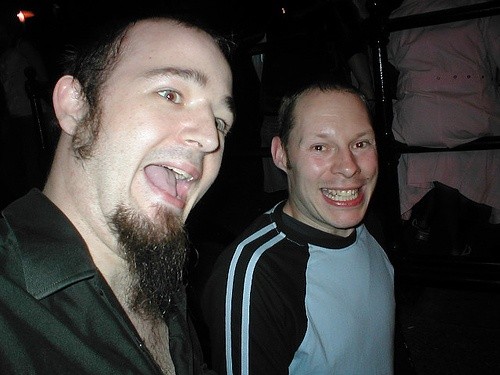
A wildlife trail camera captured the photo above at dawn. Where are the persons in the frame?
[196,74,403,372]
[0,13,238,374]
